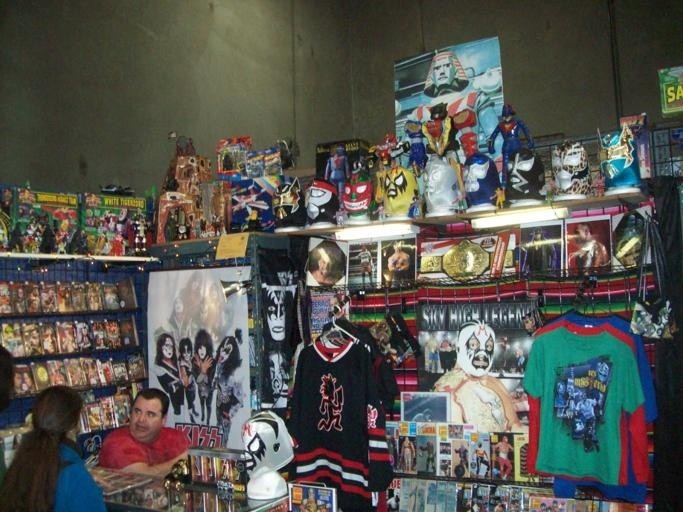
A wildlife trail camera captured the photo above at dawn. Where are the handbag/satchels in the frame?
[628,298,678,340]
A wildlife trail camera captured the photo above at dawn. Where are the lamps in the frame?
[218,278,253,303]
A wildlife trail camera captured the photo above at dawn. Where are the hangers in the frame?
[320,293,372,353]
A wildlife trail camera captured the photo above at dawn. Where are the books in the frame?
[288,483,338,512]
[388,432,628,512]
[1,281,148,471]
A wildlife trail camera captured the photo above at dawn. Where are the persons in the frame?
[369,104,542,220]
[348,244,375,284]
[520,228,556,272]
[101,387,195,479]
[4,386,111,512]
[386,242,411,287]
[618,213,642,253]
[569,223,610,273]
[431,322,519,430]
